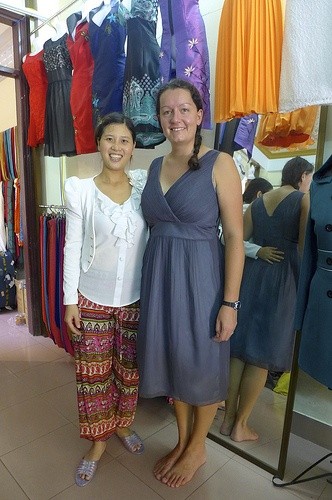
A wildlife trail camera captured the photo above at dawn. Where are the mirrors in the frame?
[207,105,327,481]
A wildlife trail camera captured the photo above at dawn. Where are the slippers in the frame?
[74,452,105,486]
[115,431,144,455]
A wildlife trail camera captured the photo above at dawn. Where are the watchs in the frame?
[222,300,242,311]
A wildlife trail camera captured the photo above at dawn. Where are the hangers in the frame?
[40,205,66,220]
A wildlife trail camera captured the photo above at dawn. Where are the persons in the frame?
[20,0,332,157]
[164,157,314,442]
[61,112,152,488]
[135,79,244,487]
[295,157,332,392]
